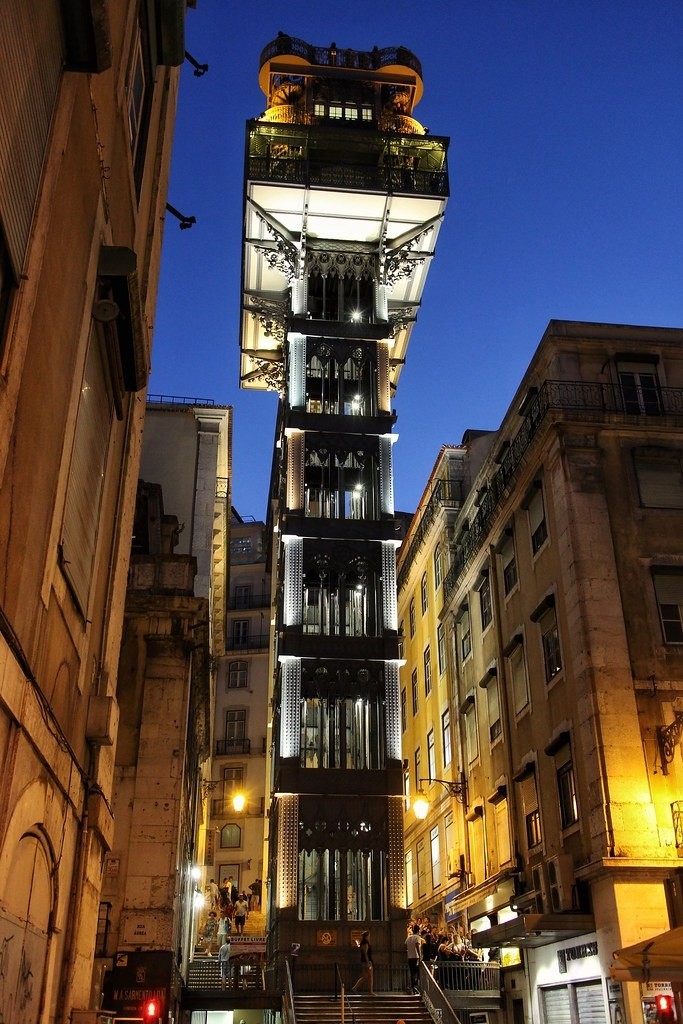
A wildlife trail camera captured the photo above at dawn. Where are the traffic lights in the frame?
[143,999,159,1024]
[656,995,672,1020]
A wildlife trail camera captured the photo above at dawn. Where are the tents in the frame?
[609,926,682,982]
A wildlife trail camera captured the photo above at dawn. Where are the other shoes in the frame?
[413,986,420,993]
[207,952,212,957]
[369,993,377,996]
[352,988,358,994]
[205,949,208,953]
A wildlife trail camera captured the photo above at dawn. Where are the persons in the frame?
[353,932,376,996]
[405,928,423,993]
[215,911,232,947]
[204,912,219,956]
[207,876,263,933]
[218,936,232,992]
[407,906,500,990]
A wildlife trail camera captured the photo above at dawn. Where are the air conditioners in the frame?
[601,447,634,484]
[544,852,574,913]
[531,862,553,914]
[446,848,461,877]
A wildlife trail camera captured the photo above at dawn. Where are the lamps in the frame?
[412,779,465,819]
[202,778,245,812]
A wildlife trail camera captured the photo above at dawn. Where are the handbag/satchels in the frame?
[226,918,231,933]
